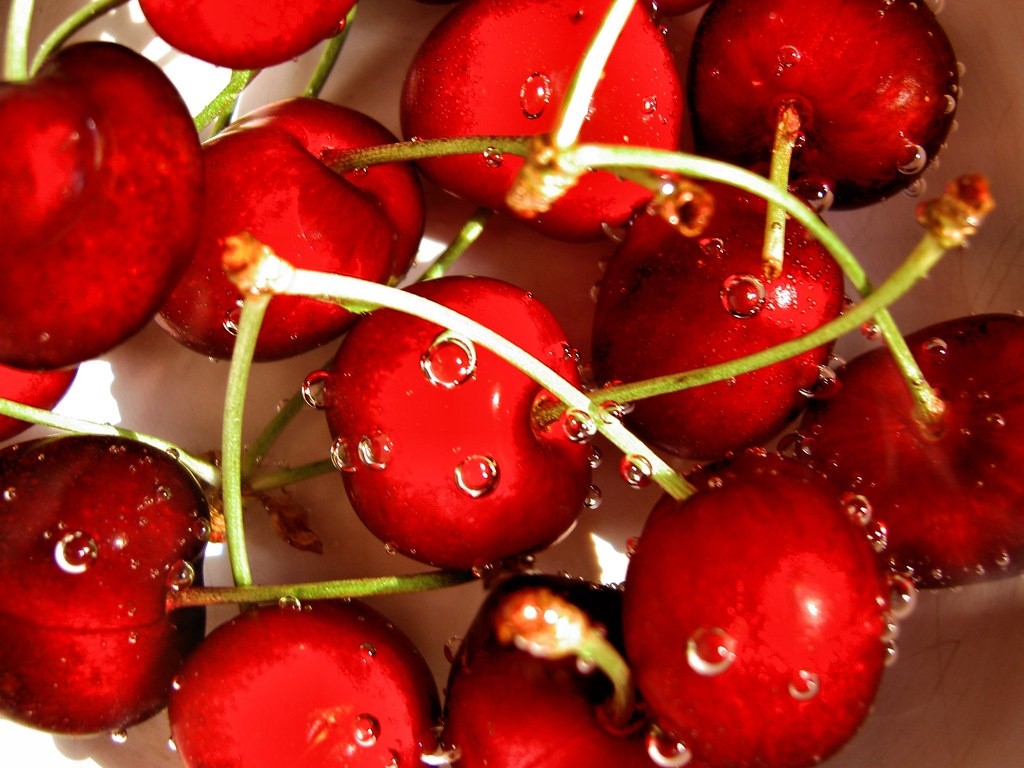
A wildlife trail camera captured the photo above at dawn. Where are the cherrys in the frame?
[0,0,1024,768]
